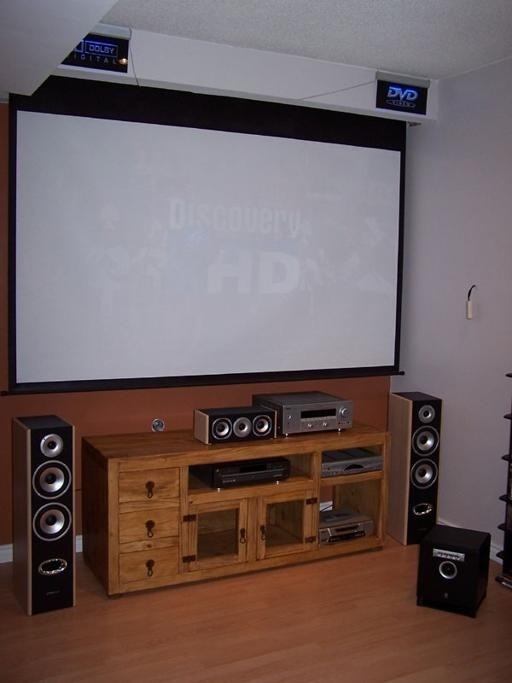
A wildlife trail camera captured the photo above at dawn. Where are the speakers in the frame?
[11,414,76,616]
[385,392,442,546]
[193,405,278,444]
[416,525,491,618]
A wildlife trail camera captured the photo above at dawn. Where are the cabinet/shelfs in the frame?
[495,371,512,590]
